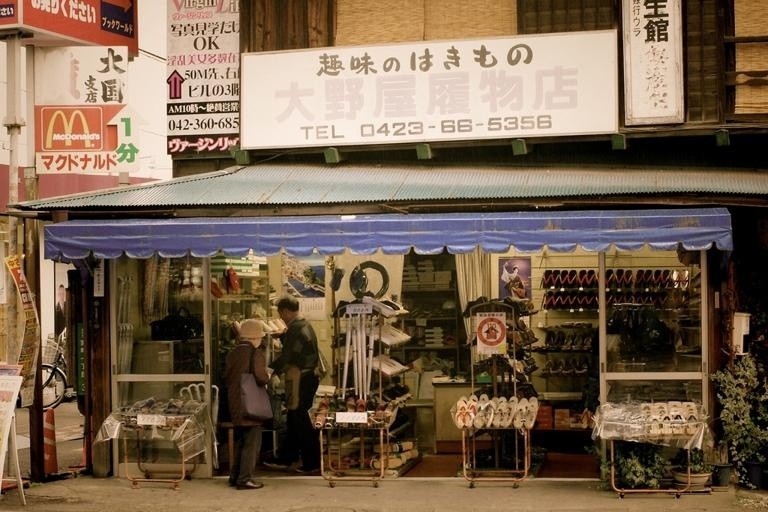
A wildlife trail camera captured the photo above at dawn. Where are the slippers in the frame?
[370,441,418,470]
[539,268,678,308]
[640,401,699,437]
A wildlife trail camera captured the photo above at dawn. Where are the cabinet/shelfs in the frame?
[131,334,221,451]
[178,268,273,378]
[535,270,692,316]
[258,326,294,458]
[428,377,502,456]
[525,337,597,455]
[459,297,540,434]
[309,295,413,433]
[395,282,462,453]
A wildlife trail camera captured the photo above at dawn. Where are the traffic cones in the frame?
[19,405,72,482]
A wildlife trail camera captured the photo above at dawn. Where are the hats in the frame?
[238,319,267,340]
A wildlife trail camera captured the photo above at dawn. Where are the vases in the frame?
[711,462,733,489]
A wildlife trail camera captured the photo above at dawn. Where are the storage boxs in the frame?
[210,247,256,274]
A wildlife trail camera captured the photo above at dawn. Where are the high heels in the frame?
[540,330,593,373]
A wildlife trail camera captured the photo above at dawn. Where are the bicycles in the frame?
[43,326,69,413]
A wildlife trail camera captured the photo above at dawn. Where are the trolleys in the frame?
[320,305,392,490]
[456,301,535,490]
[112,402,211,492]
[595,400,702,500]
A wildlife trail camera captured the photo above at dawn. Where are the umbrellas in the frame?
[342,312,378,403]
[179,383,219,469]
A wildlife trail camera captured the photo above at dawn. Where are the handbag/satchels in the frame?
[149,306,202,341]
[606,310,632,334]
[239,372,275,420]
[632,305,675,360]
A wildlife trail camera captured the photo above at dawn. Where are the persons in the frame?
[263,295,324,474]
[223,320,281,490]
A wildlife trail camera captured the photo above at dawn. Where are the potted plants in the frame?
[668,444,713,489]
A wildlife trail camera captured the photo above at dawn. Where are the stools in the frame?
[215,417,238,468]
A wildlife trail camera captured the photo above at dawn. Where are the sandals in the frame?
[506,295,539,375]
[314,395,397,428]
[453,393,538,430]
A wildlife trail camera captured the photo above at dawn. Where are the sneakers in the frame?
[228,477,239,486]
[296,464,320,473]
[263,459,289,471]
[237,480,264,490]
